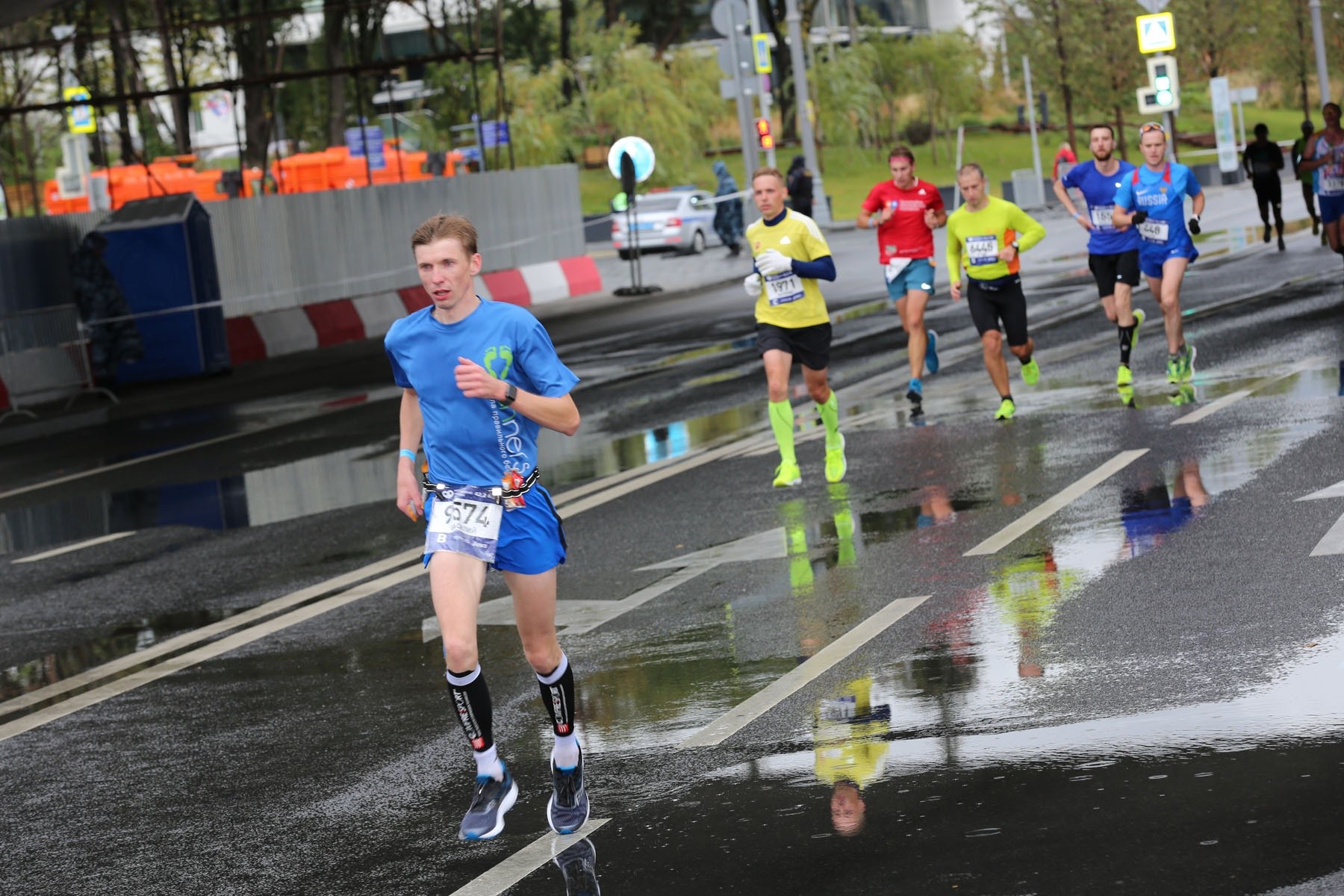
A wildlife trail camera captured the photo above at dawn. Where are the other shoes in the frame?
[1313,217,1321,235]
[1322,235,1328,246]
[1263,224,1272,243]
[1278,237,1285,251]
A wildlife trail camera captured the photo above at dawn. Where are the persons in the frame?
[383,209,588,842]
[711,159,746,258]
[947,162,1048,420]
[1242,103,1344,260]
[776,404,1214,840]
[1111,120,1205,384]
[1053,126,1146,385]
[785,153,815,219]
[857,146,948,402]
[742,168,848,487]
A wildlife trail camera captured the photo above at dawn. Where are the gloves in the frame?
[755,248,792,277]
[744,273,763,296]
[1131,210,1148,224]
[1188,219,1200,235]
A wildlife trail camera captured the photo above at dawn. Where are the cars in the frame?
[609,187,725,261]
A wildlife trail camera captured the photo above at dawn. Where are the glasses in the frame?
[1139,121,1164,136]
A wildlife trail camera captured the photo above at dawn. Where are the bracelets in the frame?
[1074,212,1081,219]
[399,449,416,463]
[869,217,873,229]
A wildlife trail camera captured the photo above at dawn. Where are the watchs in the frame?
[1010,240,1019,252]
[499,380,517,405]
[1192,213,1200,222]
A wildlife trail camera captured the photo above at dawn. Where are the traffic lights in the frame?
[1146,57,1181,113]
[753,116,774,150]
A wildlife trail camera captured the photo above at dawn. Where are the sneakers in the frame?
[546,737,589,834]
[459,761,518,842]
[1021,356,1039,386]
[1116,366,1133,386]
[1167,357,1182,384]
[906,378,923,404]
[994,400,1016,420]
[1130,308,1147,348]
[925,329,940,374]
[773,459,803,488]
[1180,345,1197,383]
[825,432,847,483]
